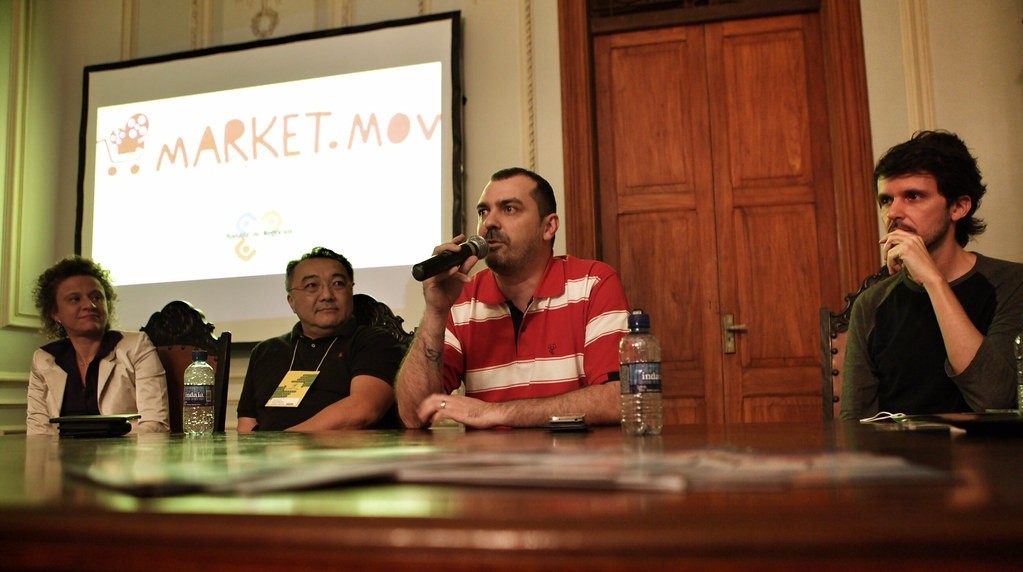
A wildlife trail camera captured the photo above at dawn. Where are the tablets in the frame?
[48,414,142,423]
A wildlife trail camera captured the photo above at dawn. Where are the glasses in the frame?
[289,276,351,295]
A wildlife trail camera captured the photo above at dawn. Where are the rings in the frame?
[440,401,446,408]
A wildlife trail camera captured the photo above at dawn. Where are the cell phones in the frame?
[546,413,585,424]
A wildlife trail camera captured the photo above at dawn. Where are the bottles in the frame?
[619,315,663,435]
[182,350,214,436]
[1014,336,1023,415]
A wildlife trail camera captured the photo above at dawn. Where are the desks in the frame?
[0,435,1023,572]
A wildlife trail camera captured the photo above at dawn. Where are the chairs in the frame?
[348,292,433,431]
[818,262,893,419]
[140,300,232,438]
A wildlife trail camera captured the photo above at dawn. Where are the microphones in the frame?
[412,234,489,282]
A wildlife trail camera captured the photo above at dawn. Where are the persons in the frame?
[838,130,1023,422]
[395,167,639,428]
[236,246,401,432]
[26,255,170,434]
